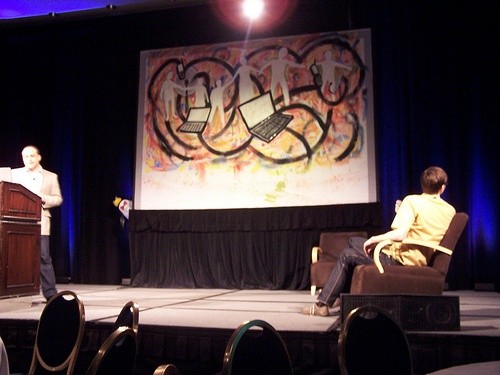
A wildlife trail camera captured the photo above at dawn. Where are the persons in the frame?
[12,146,63,303]
[301,166,456,315]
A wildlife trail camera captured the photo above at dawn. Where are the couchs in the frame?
[309,232,368,301]
[350,213,470,295]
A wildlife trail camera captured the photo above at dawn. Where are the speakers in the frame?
[340,293,461,331]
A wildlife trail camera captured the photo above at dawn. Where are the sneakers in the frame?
[301,304,330,317]
[328,297,341,312]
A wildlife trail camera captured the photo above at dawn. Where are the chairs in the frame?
[29,290,416,375]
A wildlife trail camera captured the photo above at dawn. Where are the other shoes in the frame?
[47,296,53,303]
